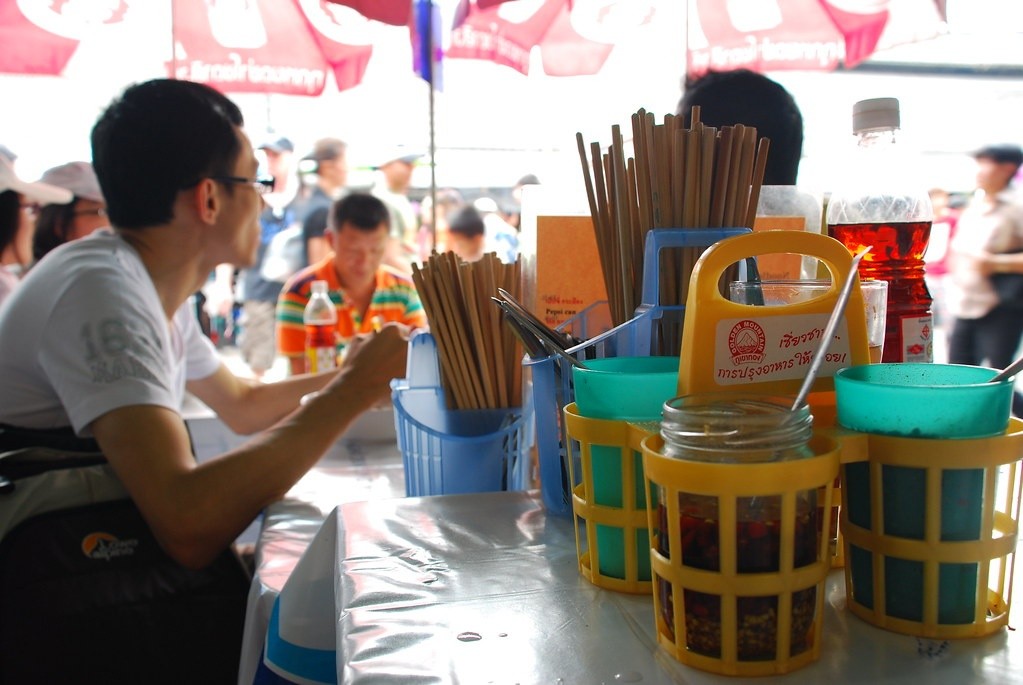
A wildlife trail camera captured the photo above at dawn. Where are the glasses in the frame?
[206,174,276,197]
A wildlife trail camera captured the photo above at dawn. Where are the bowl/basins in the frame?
[301,390,397,442]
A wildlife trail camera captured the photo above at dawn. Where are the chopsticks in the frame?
[410,250,522,408]
[576,106,770,359]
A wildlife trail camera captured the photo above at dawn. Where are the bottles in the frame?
[303,280,338,371]
[825,96,934,365]
[659,391,821,662]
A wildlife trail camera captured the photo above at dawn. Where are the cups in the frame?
[833,360,1016,624]
[571,355,682,582]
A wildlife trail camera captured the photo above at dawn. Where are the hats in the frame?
[0,145,73,204]
[257,136,293,154]
[366,140,424,170]
[301,137,347,160]
[972,144,1023,165]
[38,162,106,202]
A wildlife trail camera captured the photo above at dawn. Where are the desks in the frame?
[238,440,1023,685]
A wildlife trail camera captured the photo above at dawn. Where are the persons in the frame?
[923,143,1022,420]
[0,80,413,684]
[0,153,111,308]
[228,137,540,376]
[674,67,822,303]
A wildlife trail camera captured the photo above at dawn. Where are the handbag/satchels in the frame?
[0,435,291,685]
[987,246,1023,315]
